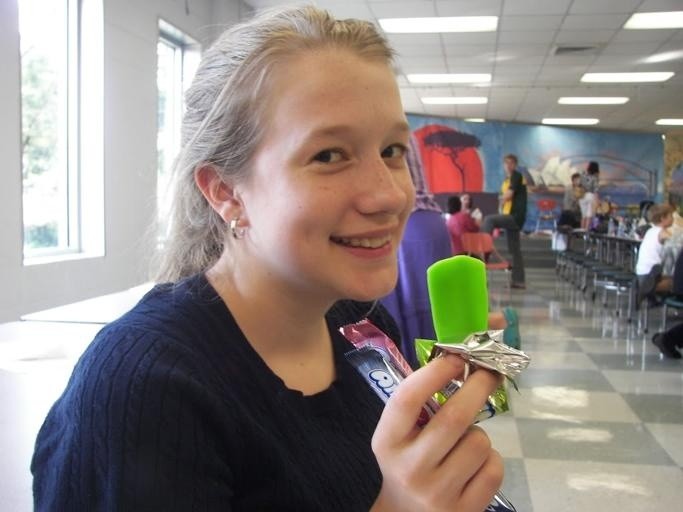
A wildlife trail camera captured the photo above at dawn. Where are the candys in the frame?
[426,255,489,343]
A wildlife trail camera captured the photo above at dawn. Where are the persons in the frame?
[558,161,683,359]
[445,196,479,256]
[479,156,527,289]
[459,193,480,219]
[29,8,505,512]
[379,131,454,371]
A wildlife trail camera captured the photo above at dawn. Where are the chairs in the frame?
[458,231,513,316]
[532,199,560,236]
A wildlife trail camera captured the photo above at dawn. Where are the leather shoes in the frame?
[506,285,527,289]
[653,331,682,360]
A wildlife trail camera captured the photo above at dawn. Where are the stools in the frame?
[553,194,680,341]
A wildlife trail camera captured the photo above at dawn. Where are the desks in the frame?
[0,319,115,510]
[20,268,157,323]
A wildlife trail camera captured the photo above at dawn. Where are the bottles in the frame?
[606,215,637,239]
